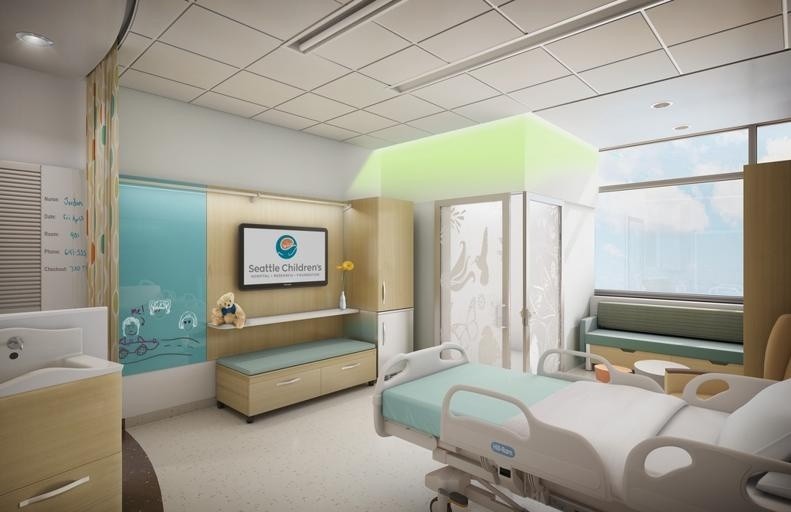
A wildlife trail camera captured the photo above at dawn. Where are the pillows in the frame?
[716,378,791,480]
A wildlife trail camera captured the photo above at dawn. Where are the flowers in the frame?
[336,260,354,291]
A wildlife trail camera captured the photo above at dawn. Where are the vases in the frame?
[338,290,346,310]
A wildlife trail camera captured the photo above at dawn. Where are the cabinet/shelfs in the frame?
[0,372,122,512]
[215,338,377,423]
[342,197,413,386]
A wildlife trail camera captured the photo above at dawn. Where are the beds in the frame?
[373,342,791,512]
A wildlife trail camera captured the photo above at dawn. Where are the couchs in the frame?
[578,296,743,376]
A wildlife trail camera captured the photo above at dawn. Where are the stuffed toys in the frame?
[211,292,247,330]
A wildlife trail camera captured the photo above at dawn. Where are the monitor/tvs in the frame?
[239,222,328,290]
